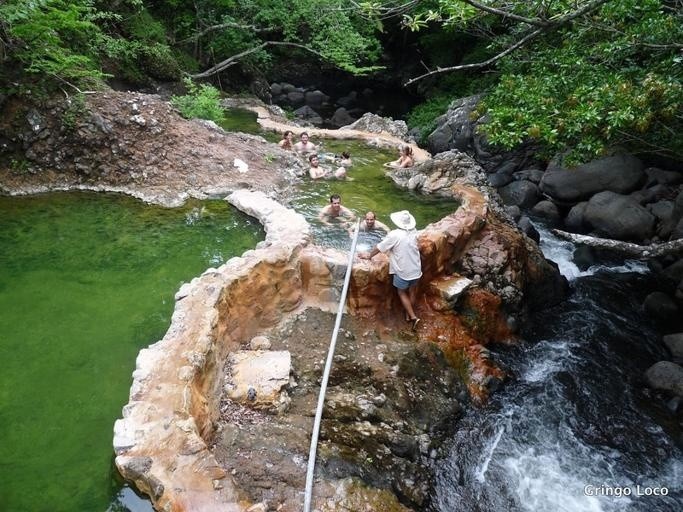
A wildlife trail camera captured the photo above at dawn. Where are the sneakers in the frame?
[411,317,420,330]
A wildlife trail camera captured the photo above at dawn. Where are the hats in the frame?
[390,209,416,229]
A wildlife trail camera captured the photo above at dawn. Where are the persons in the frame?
[347,211,391,238]
[319,194,355,227]
[278,130,353,182]
[383,144,413,170]
[357,210,423,330]
